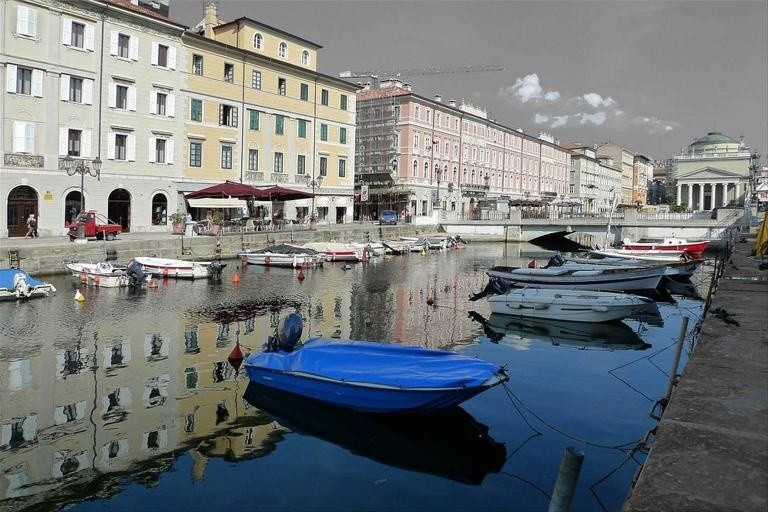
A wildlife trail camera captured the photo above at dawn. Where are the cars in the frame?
[379,210,398,226]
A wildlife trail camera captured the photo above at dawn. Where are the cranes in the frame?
[338,64,508,92]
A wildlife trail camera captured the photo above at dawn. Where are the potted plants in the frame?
[169,212,187,233]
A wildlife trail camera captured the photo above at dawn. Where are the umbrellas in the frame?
[253,184,315,215]
[182,180,263,216]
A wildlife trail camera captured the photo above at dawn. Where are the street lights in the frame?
[436,165,442,205]
[747,149,762,204]
[303,174,324,220]
[524,191,530,217]
[63,152,101,240]
[560,194,565,214]
[483,173,491,206]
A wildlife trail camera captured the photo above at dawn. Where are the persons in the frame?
[24,213,37,239]
[255,205,259,217]
[399,209,405,223]
[273,208,282,219]
[259,205,266,216]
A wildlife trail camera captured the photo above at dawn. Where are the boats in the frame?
[0,269,56,300]
[238,233,467,267]
[131,257,226,278]
[245,337,512,415]
[468,311,654,353]
[485,237,711,322]
[66,262,152,287]
[238,383,508,487]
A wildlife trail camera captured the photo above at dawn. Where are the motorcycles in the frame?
[67,210,122,241]
